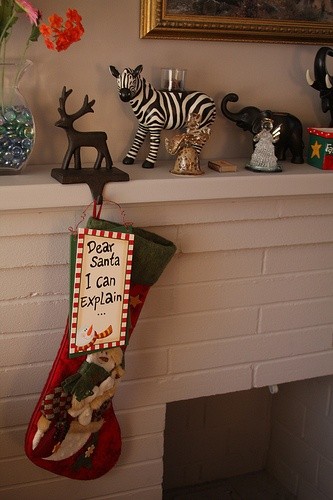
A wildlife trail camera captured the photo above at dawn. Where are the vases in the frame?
[0,57,39,173]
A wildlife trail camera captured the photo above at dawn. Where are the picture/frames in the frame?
[136,0,333,47]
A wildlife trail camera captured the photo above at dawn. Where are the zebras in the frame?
[108,64,216,168]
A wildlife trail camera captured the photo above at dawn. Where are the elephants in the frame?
[220,92,304,163]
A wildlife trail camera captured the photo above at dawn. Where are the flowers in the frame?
[0,1,84,80]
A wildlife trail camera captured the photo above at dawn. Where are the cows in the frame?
[305,46,333,128]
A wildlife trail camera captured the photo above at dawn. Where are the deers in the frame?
[53,85,112,170]
